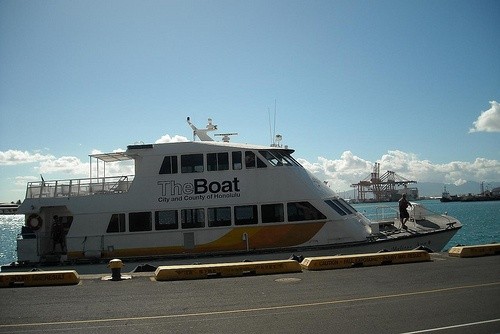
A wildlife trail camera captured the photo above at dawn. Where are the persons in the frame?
[47,215,67,253]
[399,194,413,230]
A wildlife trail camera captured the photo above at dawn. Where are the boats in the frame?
[0,117,462,272]
